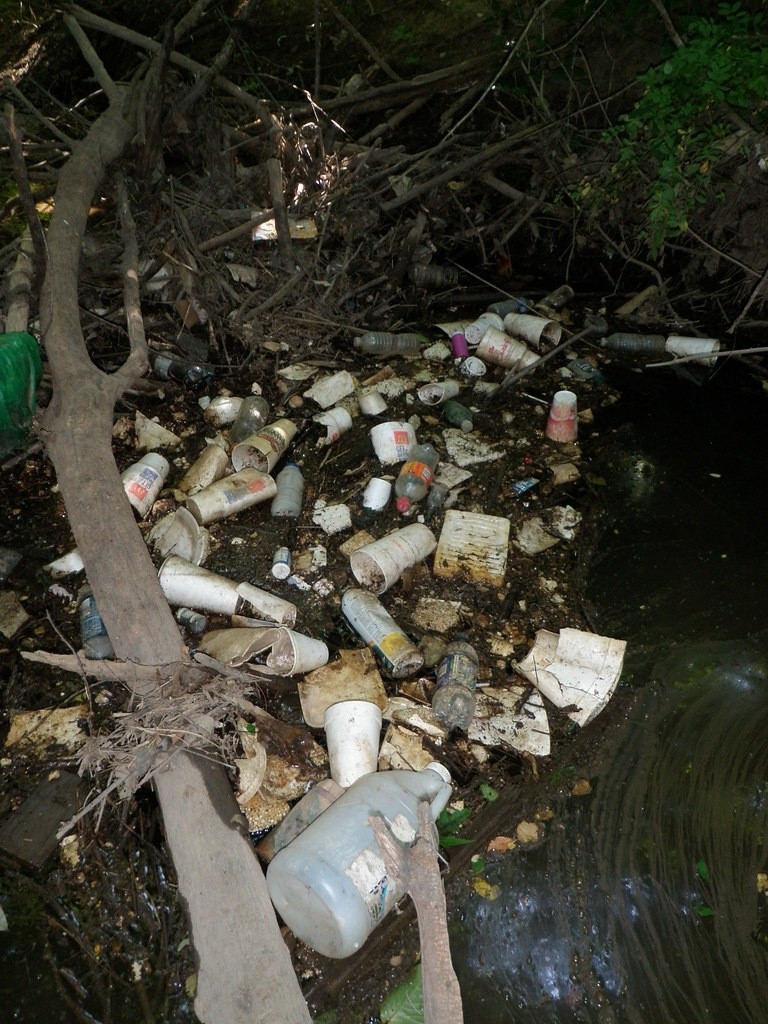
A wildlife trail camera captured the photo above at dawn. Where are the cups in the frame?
[272,627,329,678]
[501,313,561,348]
[358,389,388,416]
[233,418,298,475]
[418,382,459,405]
[544,391,579,444]
[349,522,438,594]
[665,336,719,367]
[363,478,392,512]
[324,699,382,788]
[476,325,539,374]
[120,453,169,521]
[464,313,504,344]
[302,408,353,448]
[158,556,245,617]
[186,468,278,526]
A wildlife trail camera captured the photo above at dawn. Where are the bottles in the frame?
[601,333,667,356]
[488,298,533,317]
[265,761,453,960]
[79,598,114,661]
[433,633,479,731]
[272,463,302,517]
[395,443,439,512]
[353,332,419,356]
[229,396,268,451]
[150,350,213,391]
